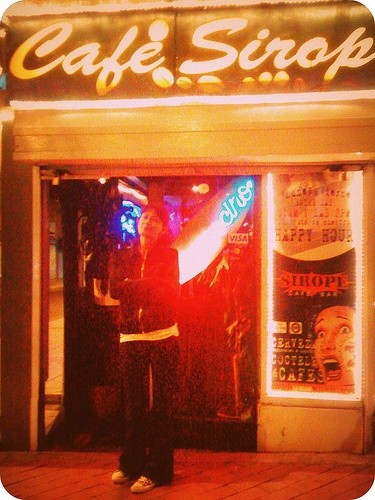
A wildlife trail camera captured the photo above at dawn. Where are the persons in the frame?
[308,300,355,394]
[109,204,180,492]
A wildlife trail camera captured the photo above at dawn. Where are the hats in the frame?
[142,203,170,223]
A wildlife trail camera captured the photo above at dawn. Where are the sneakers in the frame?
[130,472,155,494]
[112,469,130,484]
